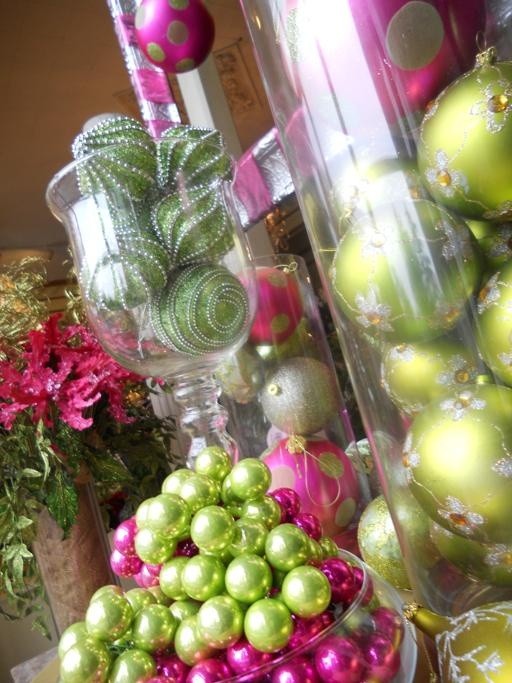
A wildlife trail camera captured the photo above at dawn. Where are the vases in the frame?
[27,449,117,642]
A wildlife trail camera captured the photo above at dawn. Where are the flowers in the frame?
[0,306,197,641]
